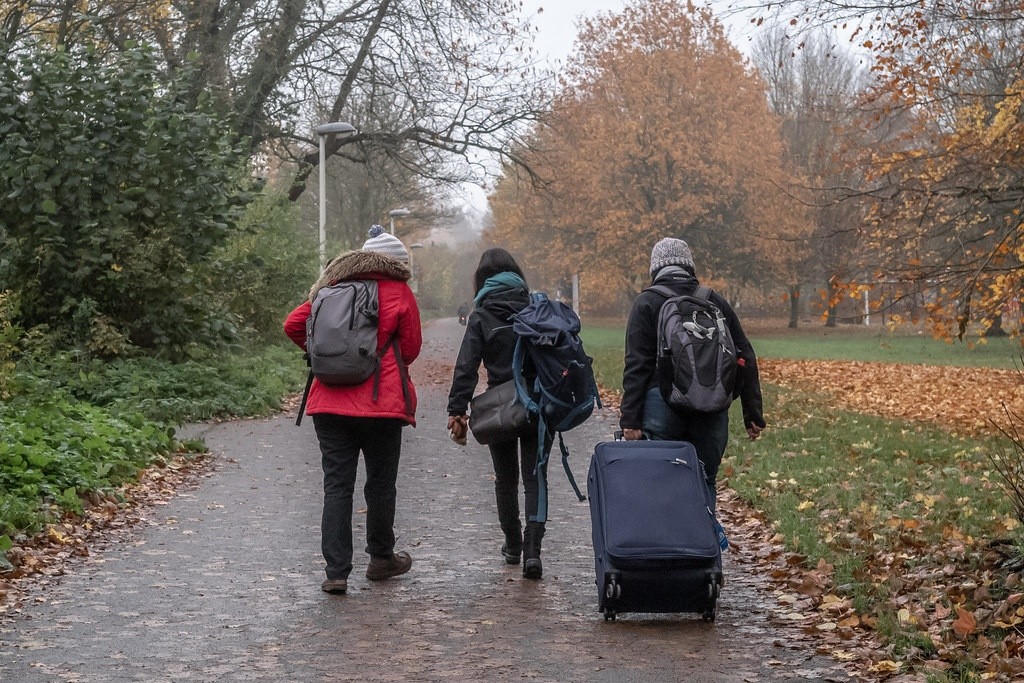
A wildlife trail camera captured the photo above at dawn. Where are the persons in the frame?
[620,239,765,518]
[284,225,424,590]
[458,300,468,326]
[448,246,557,579]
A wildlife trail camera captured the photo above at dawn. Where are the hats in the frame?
[362,225,409,262]
[650,237,696,275]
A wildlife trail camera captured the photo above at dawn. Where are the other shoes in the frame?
[366,551,412,579]
[322,578,347,590]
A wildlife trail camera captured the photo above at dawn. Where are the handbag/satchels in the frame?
[470,376,538,445]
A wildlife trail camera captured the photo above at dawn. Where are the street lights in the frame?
[388,210,411,236]
[410,243,425,283]
[316,122,356,280]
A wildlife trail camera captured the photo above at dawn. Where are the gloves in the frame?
[448,416,471,446]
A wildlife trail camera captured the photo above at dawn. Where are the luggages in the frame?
[588,431,723,621]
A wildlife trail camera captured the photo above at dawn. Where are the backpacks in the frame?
[302,275,400,384]
[642,284,737,412]
[513,293,594,431]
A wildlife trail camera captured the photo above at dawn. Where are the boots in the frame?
[524,525,544,578]
[501,520,522,563]
